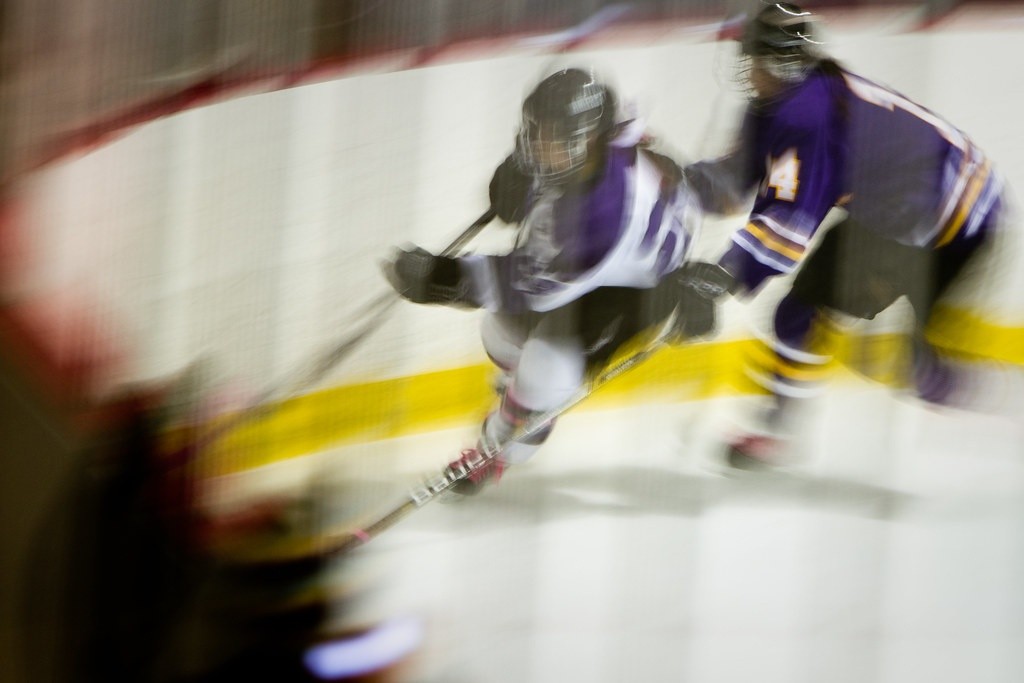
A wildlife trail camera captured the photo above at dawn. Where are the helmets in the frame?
[735,3,823,109]
[512,69,616,188]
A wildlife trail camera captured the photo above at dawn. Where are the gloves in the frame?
[394,247,484,310]
[489,150,534,224]
[655,260,733,346]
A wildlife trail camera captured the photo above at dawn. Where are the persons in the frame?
[668,2,1005,471]
[381,63,705,491]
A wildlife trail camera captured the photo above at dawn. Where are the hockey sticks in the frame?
[330,326,676,560]
[169,205,500,471]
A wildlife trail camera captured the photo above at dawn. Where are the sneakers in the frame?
[727,427,794,474]
[444,442,511,500]
